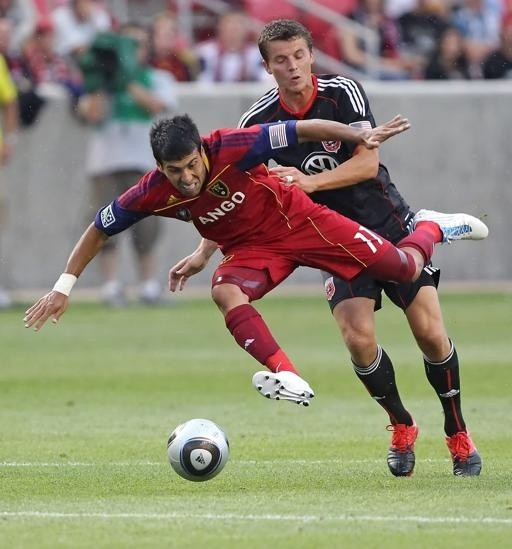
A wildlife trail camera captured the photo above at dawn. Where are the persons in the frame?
[23,114,489,405]
[0,54,20,306]
[0,0,511,128]
[169,19,482,476]
[72,21,176,305]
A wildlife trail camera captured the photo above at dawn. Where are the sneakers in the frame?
[385,416,419,477]
[251,371,315,407]
[102,282,164,306]
[444,426,482,476]
[412,209,489,244]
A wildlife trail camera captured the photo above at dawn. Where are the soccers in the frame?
[167,418,231,481]
